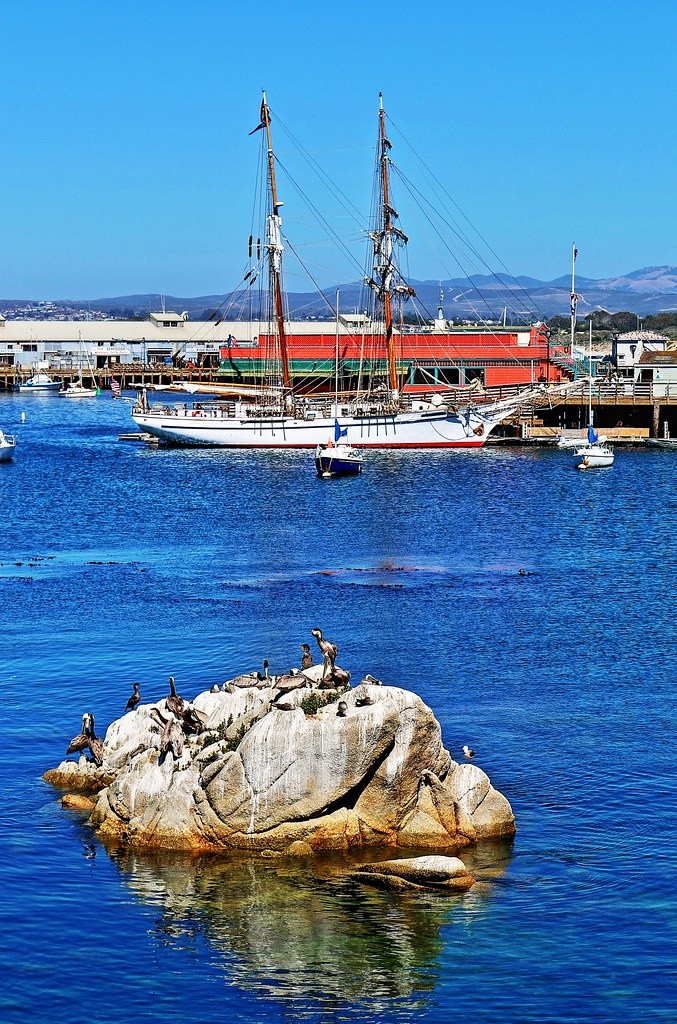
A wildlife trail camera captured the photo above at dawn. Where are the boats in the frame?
[0,426,17,463]
[11,358,65,392]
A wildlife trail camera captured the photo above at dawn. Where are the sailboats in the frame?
[310,287,365,480]
[57,330,101,399]
[573,320,615,471]
[122,84,603,451]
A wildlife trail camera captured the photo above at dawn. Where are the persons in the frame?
[227,334,238,347]
[253,336,259,347]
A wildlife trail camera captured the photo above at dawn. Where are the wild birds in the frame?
[358,674,382,686]
[87,714,103,766]
[462,746,476,764]
[66,713,91,755]
[209,680,235,693]
[182,705,209,736]
[355,685,376,707]
[300,644,312,668]
[166,677,184,720]
[124,683,140,710]
[311,628,351,688]
[337,701,348,713]
